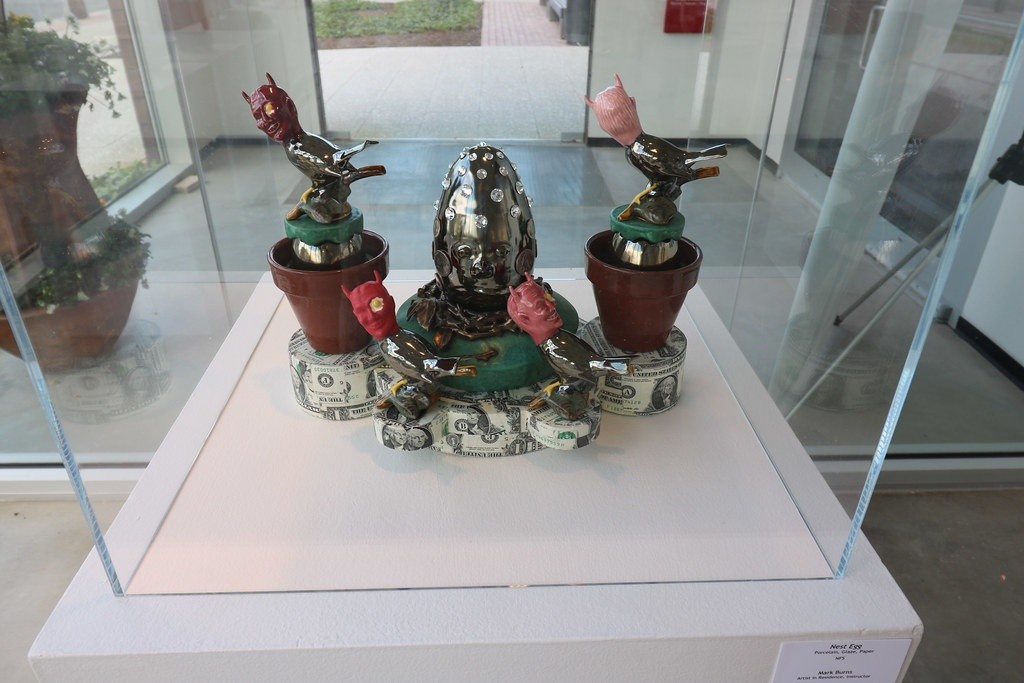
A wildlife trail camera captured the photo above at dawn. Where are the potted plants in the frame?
[0,0,153,374]
